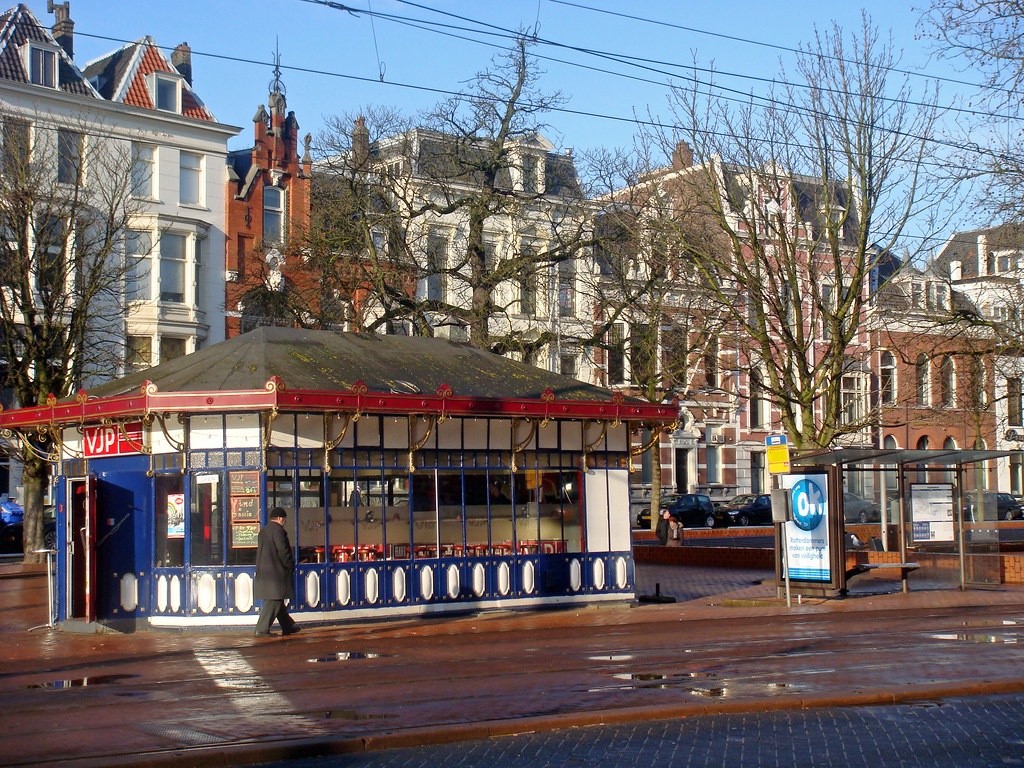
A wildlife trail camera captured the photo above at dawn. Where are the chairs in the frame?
[748,499,753,504]
[743,497,748,502]
[686,498,693,504]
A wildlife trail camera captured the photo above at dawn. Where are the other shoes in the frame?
[282,626,301,635]
[254,631,278,637]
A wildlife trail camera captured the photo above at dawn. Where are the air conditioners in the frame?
[711,434,725,443]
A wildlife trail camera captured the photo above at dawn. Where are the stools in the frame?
[310,544,540,563]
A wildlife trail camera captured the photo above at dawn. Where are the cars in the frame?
[714,494,772,526]
[0,505,56,552]
[841,492,891,524]
[637,493,717,529]
[955,491,1021,520]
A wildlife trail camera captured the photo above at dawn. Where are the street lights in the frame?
[871,309,889,554]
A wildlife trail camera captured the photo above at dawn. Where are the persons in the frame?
[656,510,671,545]
[666,517,684,547]
[255,507,301,638]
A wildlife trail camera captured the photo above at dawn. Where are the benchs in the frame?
[852,560,920,595]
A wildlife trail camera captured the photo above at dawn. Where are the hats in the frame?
[270,507,287,517]
[669,517,676,522]
